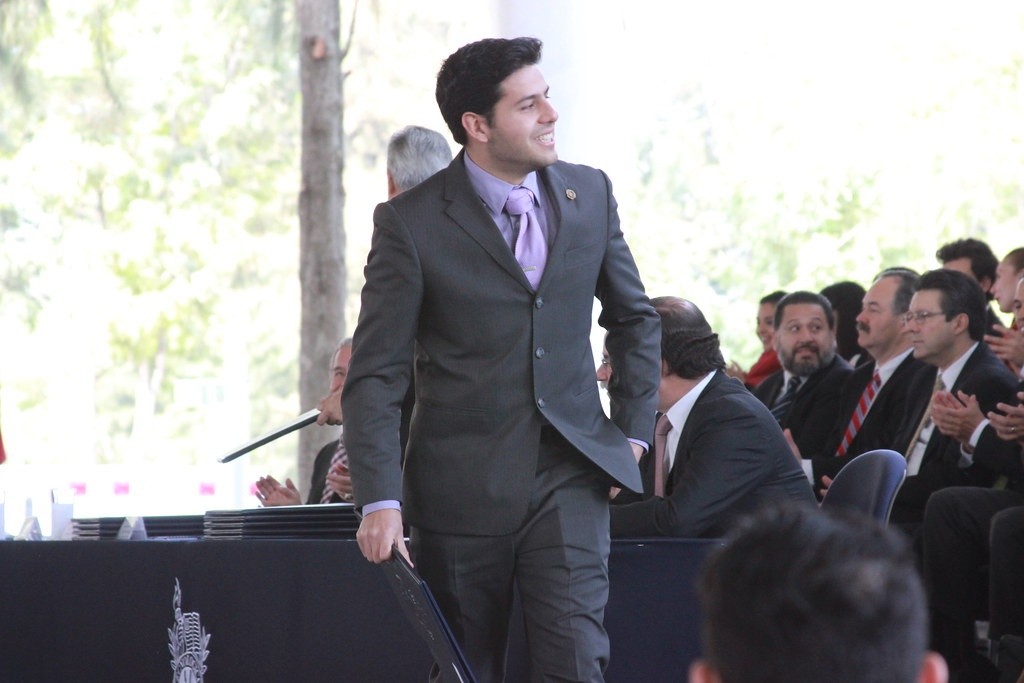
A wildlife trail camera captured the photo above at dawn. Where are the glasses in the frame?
[602,359,612,370]
[904,310,950,324]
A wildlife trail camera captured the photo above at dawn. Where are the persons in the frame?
[753,291,854,461]
[598,330,625,389]
[253,339,355,505]
[990,248,1024,312]
[389,127,452,197]
[810,268,928,509]
[935,238,999,301]
[609,295,818,538]
[983,277,1024,384]
[818,281,871,368]
[724,292,787,391]
[987,391,1024,441]
[341,39,661,683]
[904,269,1020,585]
[922,390,1024,683]
[687,502,948,683]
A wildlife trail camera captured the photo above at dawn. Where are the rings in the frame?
[1012,426,1015,432]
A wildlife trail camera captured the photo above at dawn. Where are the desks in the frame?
[0,534,731,683]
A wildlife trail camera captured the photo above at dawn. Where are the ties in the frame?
[905,379,945,464]
[319,438,348,504]
[505,187,550,295]
[769,376,801,425]
[833,373,882,457]
[655,415,673,500]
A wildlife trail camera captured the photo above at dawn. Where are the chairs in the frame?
[820,448,908,527]
